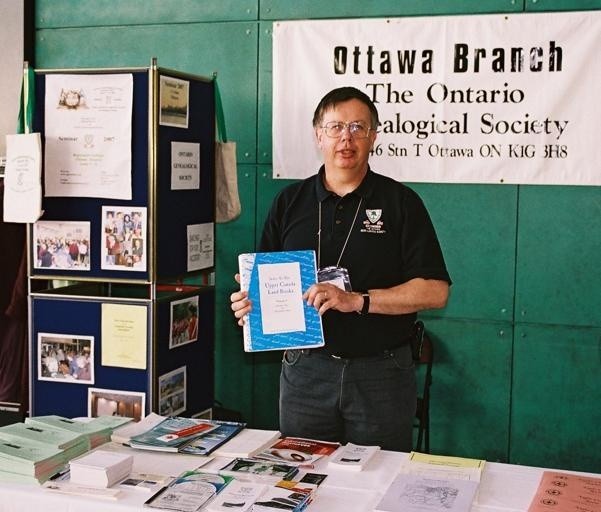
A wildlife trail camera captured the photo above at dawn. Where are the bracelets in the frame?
[355,288,372,316]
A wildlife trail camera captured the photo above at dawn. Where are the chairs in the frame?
[410,333,434,453]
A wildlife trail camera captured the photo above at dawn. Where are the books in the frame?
[237,250,327,352]
[1,408,380,512]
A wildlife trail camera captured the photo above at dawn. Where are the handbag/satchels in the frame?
[215,141,241,224]
[3,132,45,224]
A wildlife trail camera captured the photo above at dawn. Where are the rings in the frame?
[326,290,329,300]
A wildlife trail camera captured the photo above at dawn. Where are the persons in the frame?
[37,237,90,268]
[188,306,198,341]
[105,211,144,268]
[228,85,455,451]
[41,341,90,379]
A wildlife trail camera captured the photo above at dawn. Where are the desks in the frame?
[0,420,601,512]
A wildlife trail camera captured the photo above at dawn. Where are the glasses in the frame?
[320,122,372,139]
